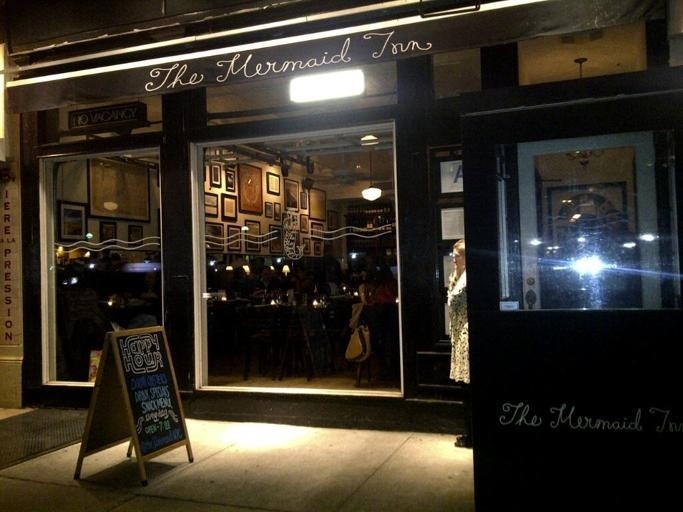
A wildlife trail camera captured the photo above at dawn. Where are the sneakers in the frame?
[455,436,469,448]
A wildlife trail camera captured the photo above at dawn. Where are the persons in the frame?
[446,238,474,448]
[89,250,137,329]
[208,250,399,386]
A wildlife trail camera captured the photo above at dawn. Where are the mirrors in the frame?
[493,129,679,315]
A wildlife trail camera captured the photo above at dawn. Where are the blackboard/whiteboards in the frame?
[110,326,189,462]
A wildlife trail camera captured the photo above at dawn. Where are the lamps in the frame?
[360,148,382,201]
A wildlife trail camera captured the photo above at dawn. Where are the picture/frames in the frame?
[55,158,328,259]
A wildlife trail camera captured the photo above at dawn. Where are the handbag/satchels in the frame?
[345,326,371,363]
